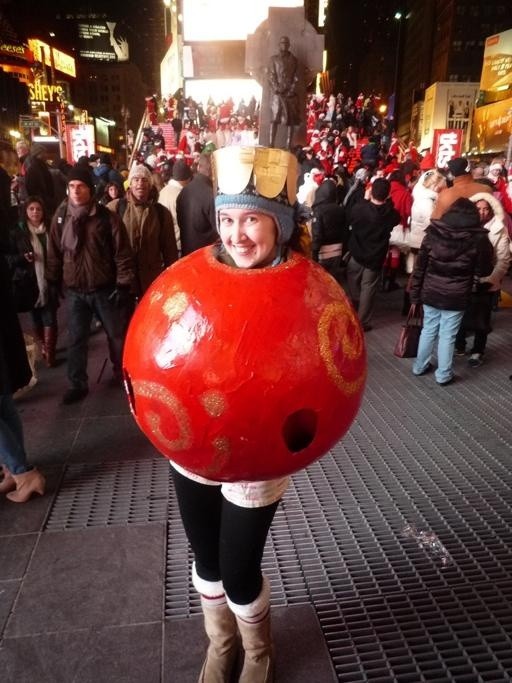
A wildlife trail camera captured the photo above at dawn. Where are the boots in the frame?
[38,319,58,367]
[0,464,16,492]
[7,466,46,503]
[191,560,242,683]
[224,572,277,683]
[383,277,400,291]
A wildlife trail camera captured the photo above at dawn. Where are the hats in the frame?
[212,172,297,244]
[172,159,193,180]
[127,165,153,190]
[448,157,472,176]
[67,158,95,192]
[29,142,47,158]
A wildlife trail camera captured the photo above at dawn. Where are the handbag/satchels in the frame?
[393,302,423,358]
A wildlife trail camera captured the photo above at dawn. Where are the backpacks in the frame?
[96,170,110,186]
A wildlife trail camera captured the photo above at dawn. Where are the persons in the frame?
[0,140,179,500]
[134,90,261,259]
[268,36,300,151]
[296,91,512,387]
[169,146,303,683]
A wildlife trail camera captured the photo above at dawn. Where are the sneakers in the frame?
[63,383,89,404]
[453,346,466,356]
[469,350,484,368]
[434,372,456,386]
[362,324,373,332]
[412,362,431,376]
